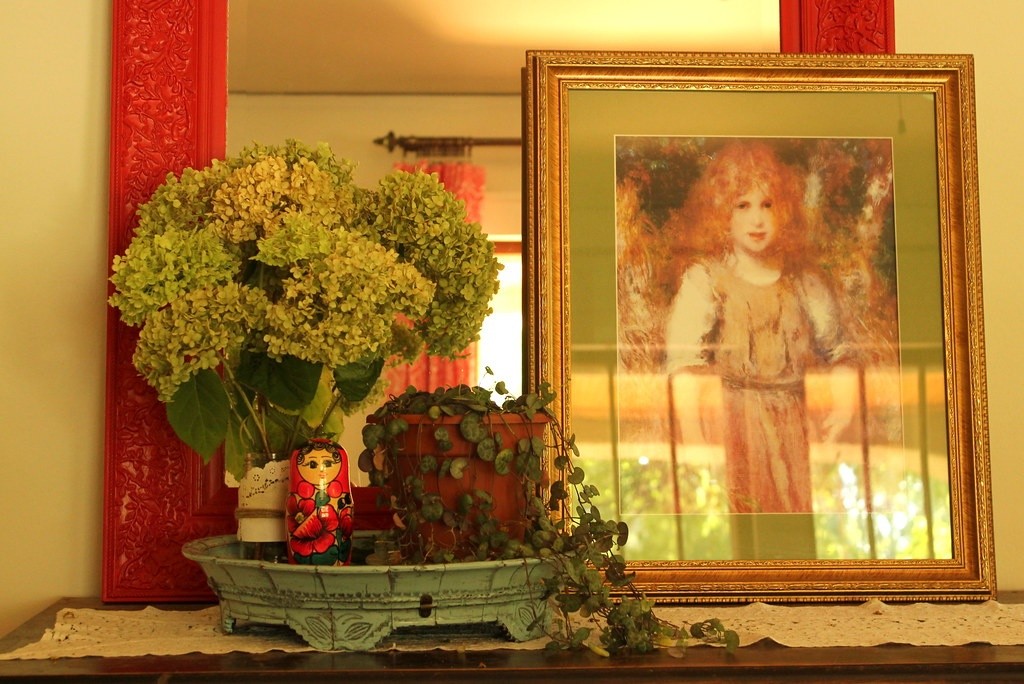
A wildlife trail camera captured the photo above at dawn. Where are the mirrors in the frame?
[101,0,896,608]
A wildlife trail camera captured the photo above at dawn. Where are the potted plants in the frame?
[351,363,742,657]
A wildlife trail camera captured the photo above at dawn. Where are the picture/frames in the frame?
[525,46,998,607]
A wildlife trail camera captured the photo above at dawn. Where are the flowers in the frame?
[105,137,505,484]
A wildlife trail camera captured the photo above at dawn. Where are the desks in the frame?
[0,591,1024,684]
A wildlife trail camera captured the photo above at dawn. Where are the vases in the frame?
[235,452,290,564]
